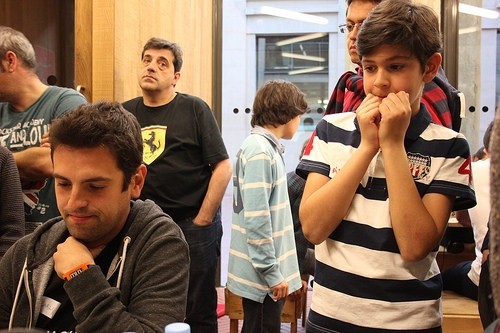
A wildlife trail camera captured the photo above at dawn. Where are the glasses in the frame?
[338,24,362,34]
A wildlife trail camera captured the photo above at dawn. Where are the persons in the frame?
[285,0,500,333]
[0,99,190,333]
[299,0,477,333]
[227,79,308,333]
[121,38,233,333]
[0,26,92,238]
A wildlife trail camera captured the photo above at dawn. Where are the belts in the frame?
[163,207,194,219]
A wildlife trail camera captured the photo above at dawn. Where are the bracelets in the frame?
[62,262,96,283]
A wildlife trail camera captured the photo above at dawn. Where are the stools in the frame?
[225,273,309,333]
